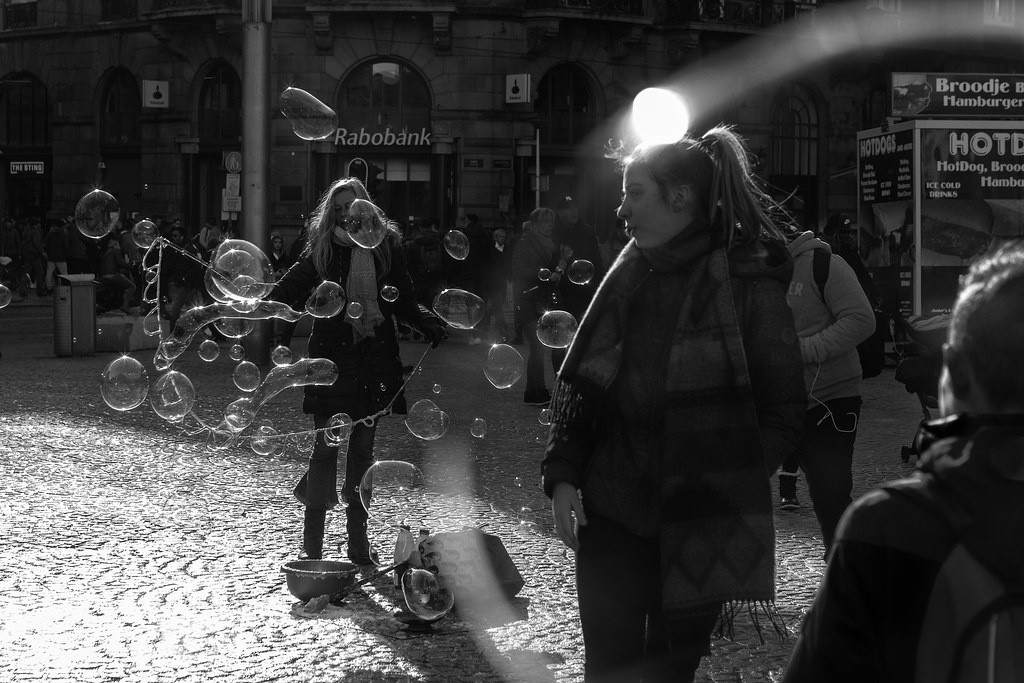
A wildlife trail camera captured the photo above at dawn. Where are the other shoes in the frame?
[524,387,554,407]
[469,337,482,345]
[120,305,134,315]
[510,337,524,345]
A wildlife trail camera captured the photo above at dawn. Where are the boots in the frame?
[296,510,332,560]
[344,506,387,560]
[777,470,800,511]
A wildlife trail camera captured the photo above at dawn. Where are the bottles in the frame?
[393,525,430,599]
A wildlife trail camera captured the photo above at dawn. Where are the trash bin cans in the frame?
[53,274,96,355]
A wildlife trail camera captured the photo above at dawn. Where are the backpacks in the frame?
[884,479,1024,683]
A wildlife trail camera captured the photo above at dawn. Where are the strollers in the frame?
[883,305,953,465]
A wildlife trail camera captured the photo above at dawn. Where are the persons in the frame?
[779,213,877,564]
[396,214,523,346]
[549,194,603,376]
[779,242,1024,683]
[538,122,809,683]
[262,175,444,575]
[512,207,566,407]
[0,216,241,341]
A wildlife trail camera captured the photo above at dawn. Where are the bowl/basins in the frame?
[281,559,360,601]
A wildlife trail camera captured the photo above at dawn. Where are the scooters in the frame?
[89,256,170,319]
